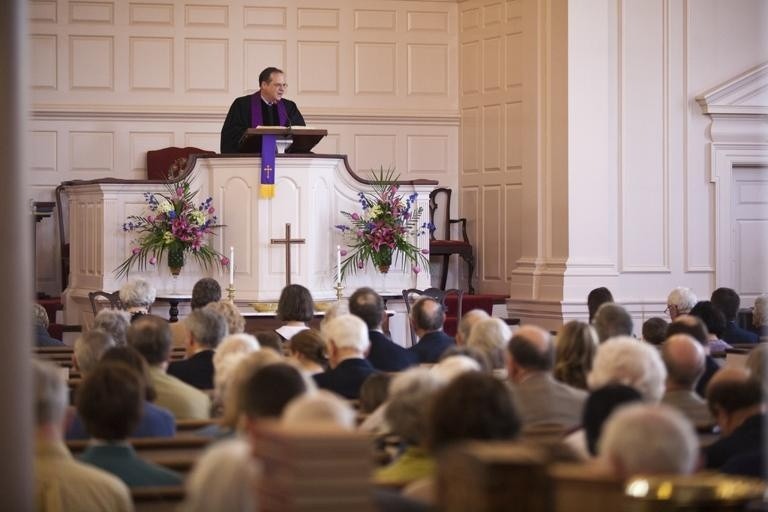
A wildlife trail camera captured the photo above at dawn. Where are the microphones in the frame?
[268,101,292,128]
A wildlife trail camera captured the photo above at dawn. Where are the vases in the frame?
[168,245,182,278]
[378,245,392,273]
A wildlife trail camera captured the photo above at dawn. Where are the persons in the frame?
[34,278,768,510]
[221,68,306,152]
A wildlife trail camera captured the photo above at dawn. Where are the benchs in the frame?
[32,343,767,511]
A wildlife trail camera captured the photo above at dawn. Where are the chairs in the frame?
[428,187,475,293]
[89,290,151,318]
[402,287,464,346]
[147,146,216,182]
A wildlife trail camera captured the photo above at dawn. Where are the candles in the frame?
[336,244,341,282]
[229,245,234,283]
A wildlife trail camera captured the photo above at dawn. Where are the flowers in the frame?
[332,164,436,283]
[112,169,230,282]
